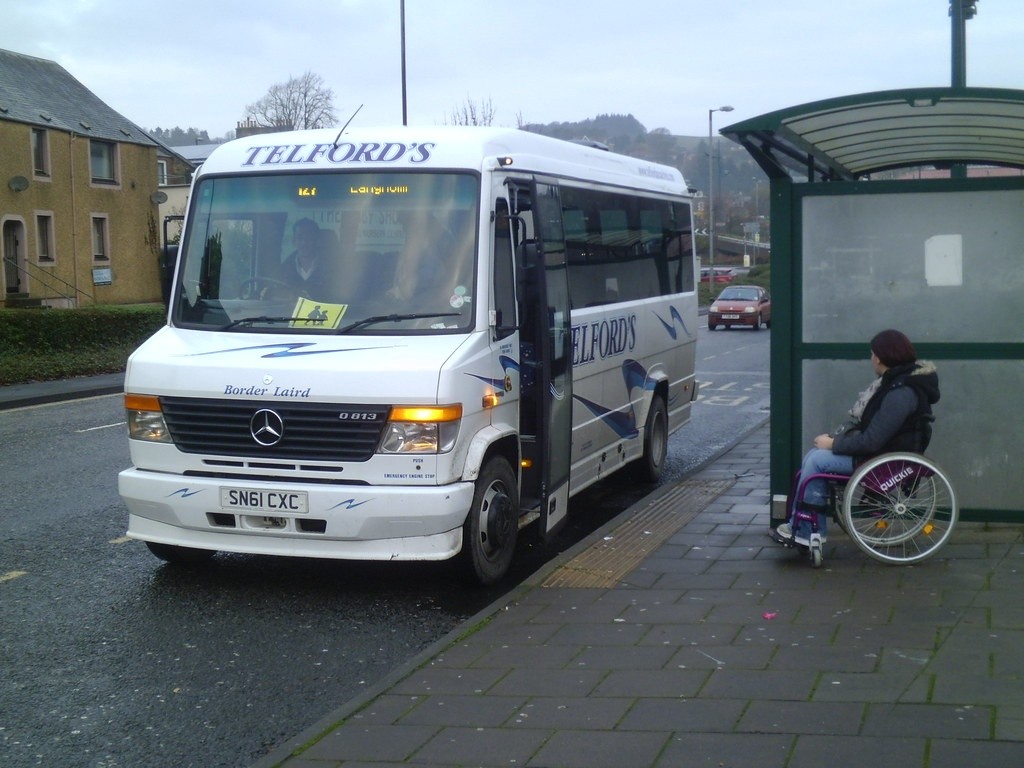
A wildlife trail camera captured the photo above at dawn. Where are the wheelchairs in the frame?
[767,449,960,569]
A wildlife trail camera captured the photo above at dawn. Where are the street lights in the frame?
[709,106,736,296]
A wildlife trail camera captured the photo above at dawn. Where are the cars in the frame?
[700,267,739,286]
[707,285,773,331]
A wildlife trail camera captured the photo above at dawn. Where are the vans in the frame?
[117,123,700,591]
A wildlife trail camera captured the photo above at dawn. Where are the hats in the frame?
[870,330,917,367]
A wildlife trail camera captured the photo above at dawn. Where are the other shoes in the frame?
[777,522,826,547]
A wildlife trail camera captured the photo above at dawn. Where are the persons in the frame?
[775,329,941,546]
[241,215,344,300]
